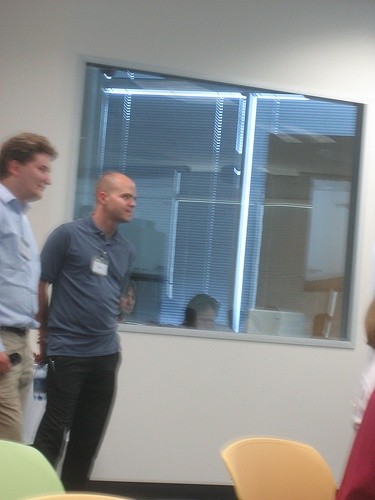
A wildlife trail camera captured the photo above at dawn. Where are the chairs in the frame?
[221,438,338,500]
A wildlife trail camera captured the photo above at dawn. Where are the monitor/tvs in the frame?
[244,309,303,336]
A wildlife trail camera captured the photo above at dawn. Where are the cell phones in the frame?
[8,352,21,365]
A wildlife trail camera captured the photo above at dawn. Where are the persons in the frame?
[31,172,138,492]
[179,294,220,327]
[119,279,138,320]
[0,132,58,443]
